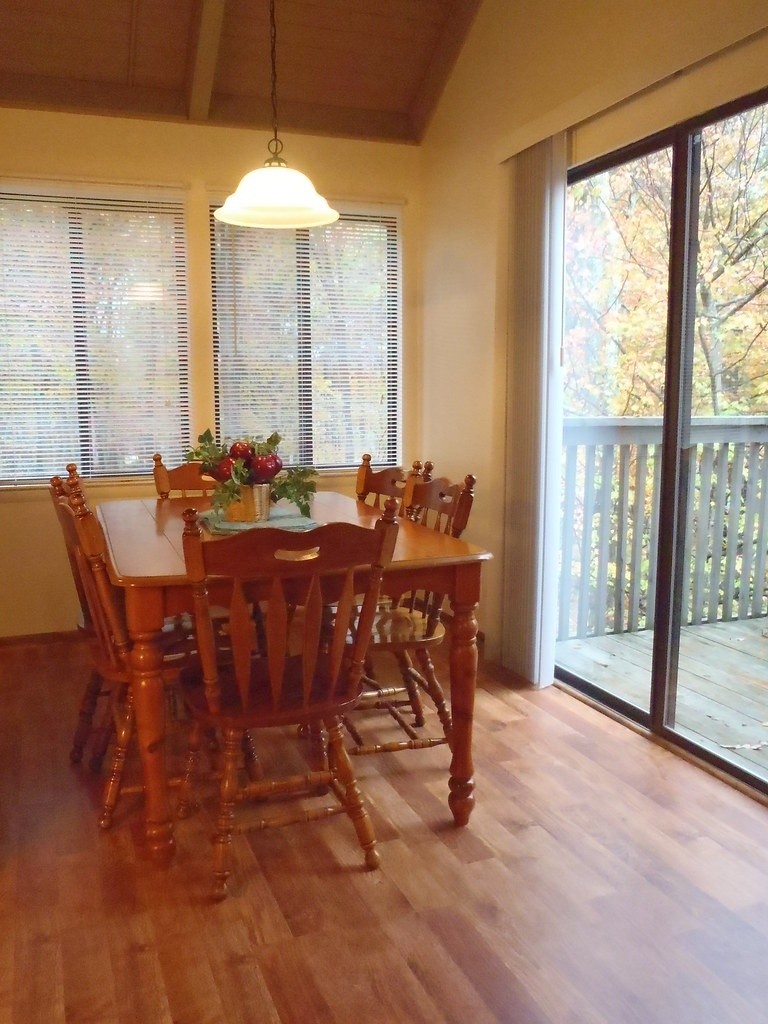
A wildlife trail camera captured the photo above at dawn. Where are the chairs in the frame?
[47,454,478,831]
[174,497,402,903]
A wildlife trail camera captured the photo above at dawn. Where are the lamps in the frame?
[213,0,340,229]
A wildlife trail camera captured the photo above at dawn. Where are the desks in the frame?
[96,491,495,869]
